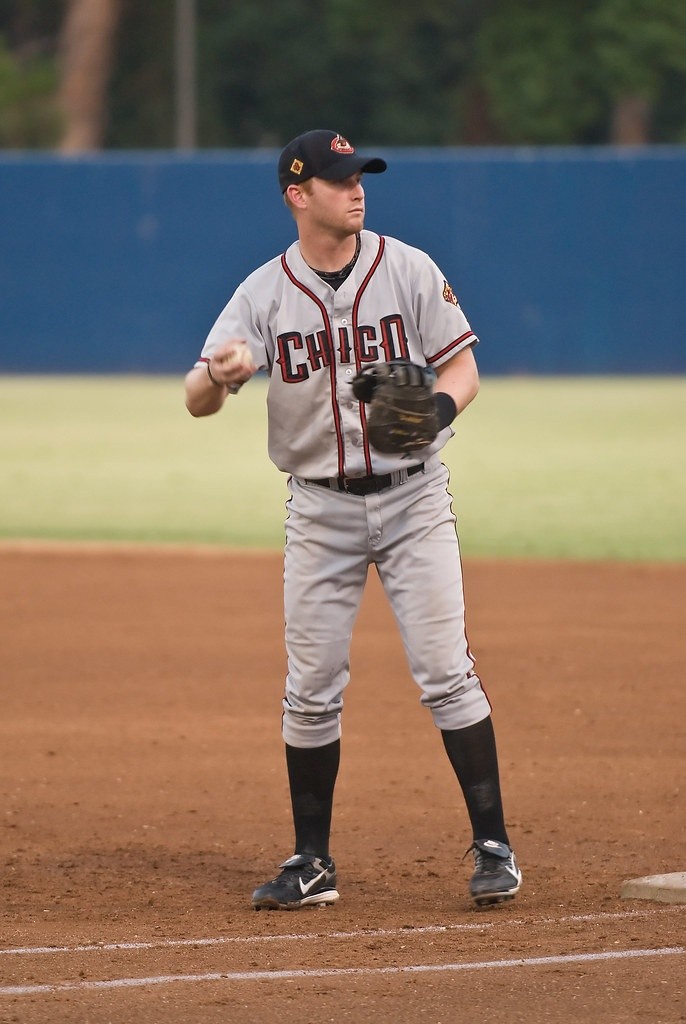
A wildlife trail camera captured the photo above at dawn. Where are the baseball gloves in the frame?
[345,361,439,455]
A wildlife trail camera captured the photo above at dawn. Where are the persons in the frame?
[183,130,523,909]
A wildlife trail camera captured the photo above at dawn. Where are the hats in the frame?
[278,130,387,194]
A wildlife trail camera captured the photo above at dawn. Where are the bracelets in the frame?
[207,365,220,387]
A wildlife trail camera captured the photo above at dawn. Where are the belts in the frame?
[306,461,425,497]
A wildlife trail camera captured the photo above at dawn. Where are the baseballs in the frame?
[221,343,251,370]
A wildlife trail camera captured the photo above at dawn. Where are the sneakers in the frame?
[250,852,339,909]
[462,839,522,905]
